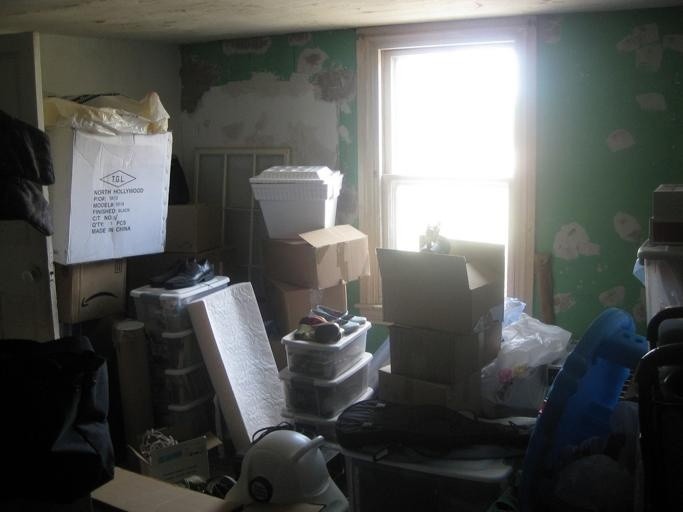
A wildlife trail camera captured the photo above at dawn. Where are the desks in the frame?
[340,445,513,512]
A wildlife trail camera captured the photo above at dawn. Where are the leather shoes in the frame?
[308,308,360,334]
[148,255,185,286]
[165,257,214,289]
[317,302,367,327]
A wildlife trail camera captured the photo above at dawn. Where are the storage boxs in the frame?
[45,125,173,267]
[146,325,203,370]
[152,361,214,405]
[386,323,503,385]
[280,386,375,451]
[280,320,372,380]
[166,202,210,254]
[248,164,344,240]
[277,351,374,419]
[54,257,128,325]
[375,241,506,334]
[260,223,372,291]
[636,237,683,346]
[263,276,348,333]
[130,275,232,333]
[377,363,484,419]
[152,391,228,436]
